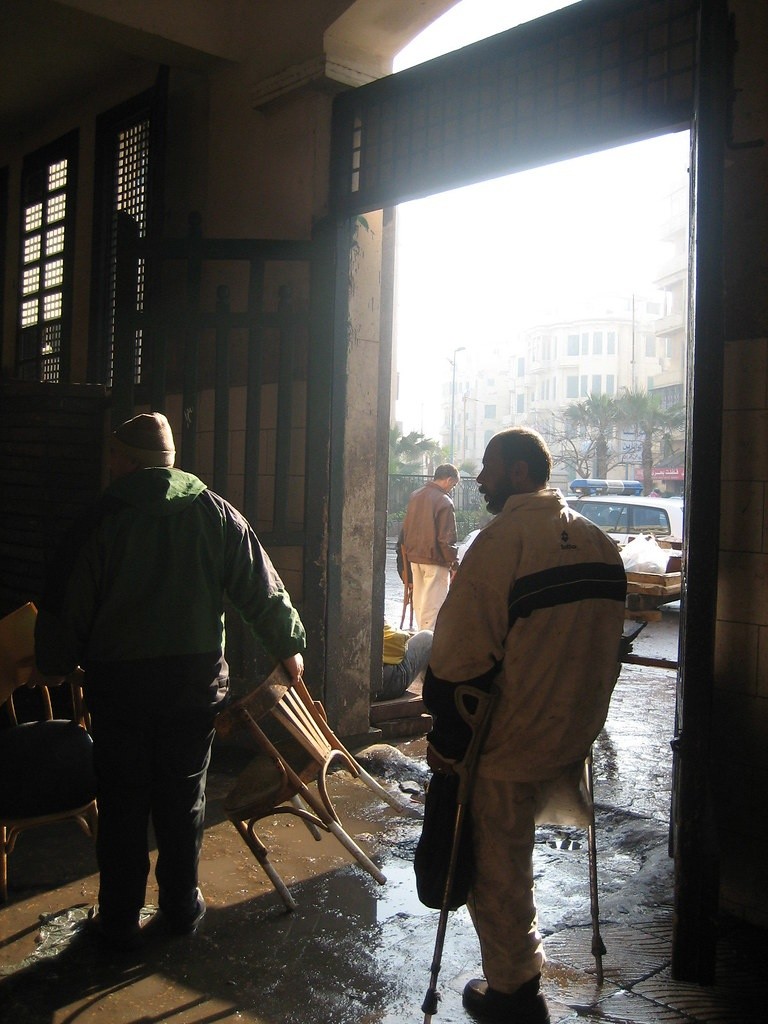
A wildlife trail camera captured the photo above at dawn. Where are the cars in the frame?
[456,479,684,609]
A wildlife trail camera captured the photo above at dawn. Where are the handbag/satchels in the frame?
[414,773,477,911]
[619,533,671,575]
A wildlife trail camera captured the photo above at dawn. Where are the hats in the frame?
[112,412,176,466]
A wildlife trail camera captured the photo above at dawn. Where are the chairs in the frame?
[215,659,402,914]
[0,601,100,905]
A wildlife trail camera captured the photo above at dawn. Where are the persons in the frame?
[421,431,627,1024]
[374,464,459,702]
[27,412,307,938]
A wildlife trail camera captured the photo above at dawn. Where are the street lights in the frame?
[450,346,467,466]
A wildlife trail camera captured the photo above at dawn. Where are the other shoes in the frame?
[91,904,142,951]
[463,979,545,1016]
[179,904,207,934]
